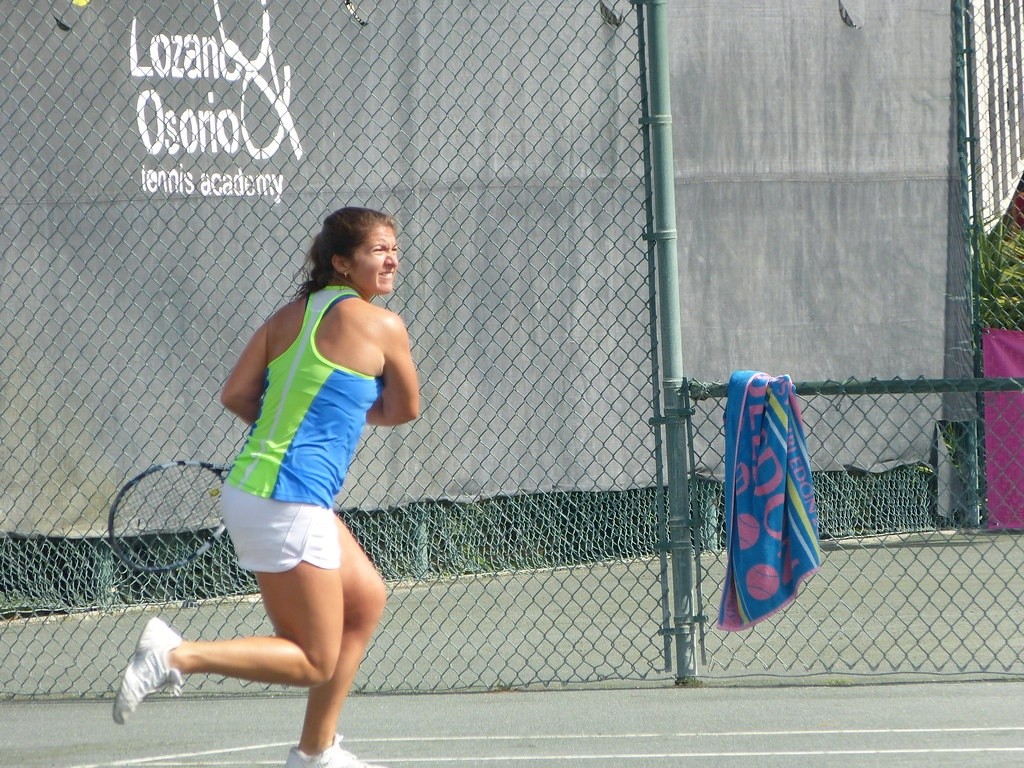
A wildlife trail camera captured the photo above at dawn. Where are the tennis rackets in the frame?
[108,461,239,573]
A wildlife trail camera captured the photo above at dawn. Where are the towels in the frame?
[709,366,824,629]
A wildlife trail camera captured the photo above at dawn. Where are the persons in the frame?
[113,205,419,768]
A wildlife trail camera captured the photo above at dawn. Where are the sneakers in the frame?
[283,733,387,768]
[113,616,183,725]
